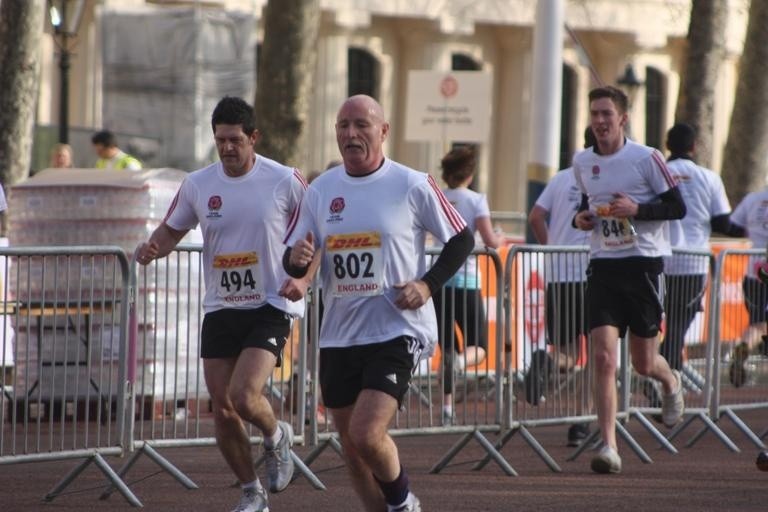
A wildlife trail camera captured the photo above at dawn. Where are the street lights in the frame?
[614,63,643,140]
[46,0,104,143]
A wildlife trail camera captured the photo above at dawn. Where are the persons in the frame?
[725,175,768,388]
[89,128,143,172]
[523,124,606,447]
[173,399,191,421]
[0,183,14,394]
[46,143,78,168]
[137,95,322,512]
[755,452,768,471]
[422,147,503,426]
[572,86,686,475]
[281,94,478,511]
[644,124,750,423]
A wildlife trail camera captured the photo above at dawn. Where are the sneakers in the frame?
[387,493,421,512]
[526,350,551,406]
[729,342,750,388]
[263,420,295,492]
[233,487,269,511]
[642,377,662,424]
[661,368,685,428]
[441,411,458,426]
[567,422,604,449]
[590,445,622,473]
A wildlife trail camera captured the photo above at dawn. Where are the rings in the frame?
[142,256,144,258]
